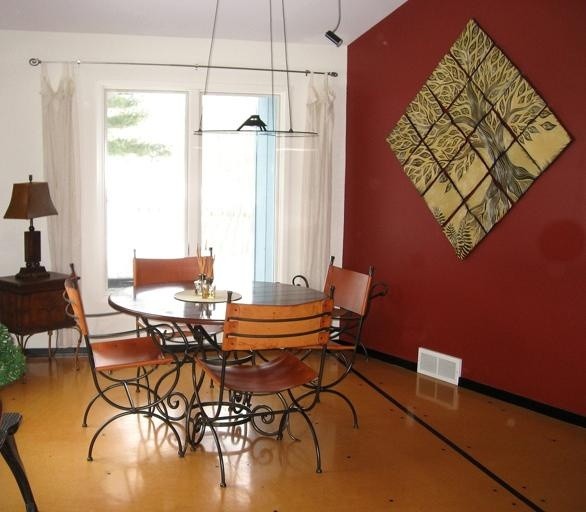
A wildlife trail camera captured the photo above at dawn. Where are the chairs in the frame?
[132,246,223,394]
[277,256,372,441]
[64,263,183,462]
[192,295,334,488]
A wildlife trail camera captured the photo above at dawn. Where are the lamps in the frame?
[193,0,353,139]
[0,173,60,278]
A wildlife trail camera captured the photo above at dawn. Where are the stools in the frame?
[0,414,39,511]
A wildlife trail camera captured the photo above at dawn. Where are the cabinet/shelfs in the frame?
[1,269,82,383]
